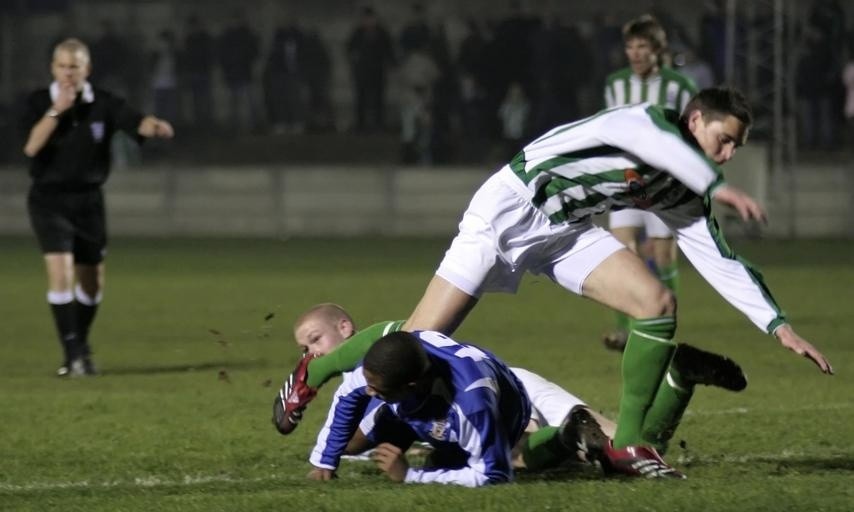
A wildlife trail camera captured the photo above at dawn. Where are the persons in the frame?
[21,37,174,380]
[597,15,706,354]
[305,327,547,491]
[0,0,854,167]
[267,80,838,483]
[291,301,745,484]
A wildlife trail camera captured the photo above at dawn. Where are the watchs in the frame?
[45,105,63,120]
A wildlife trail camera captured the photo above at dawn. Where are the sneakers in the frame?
[558,330,747,481]
[273,353,325,434]
